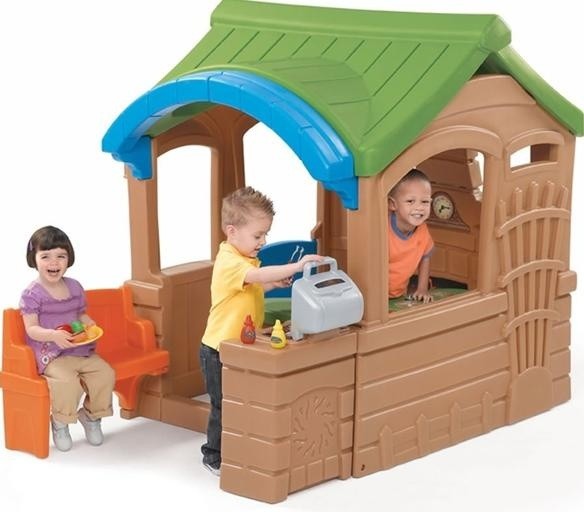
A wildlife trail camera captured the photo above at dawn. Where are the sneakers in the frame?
[203,455,223,476]
[51,414,73,451]
[77,407,105,446]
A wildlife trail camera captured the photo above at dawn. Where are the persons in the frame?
[201,186,323,479]
[385,164,436,306]
[19,223,113,451]
